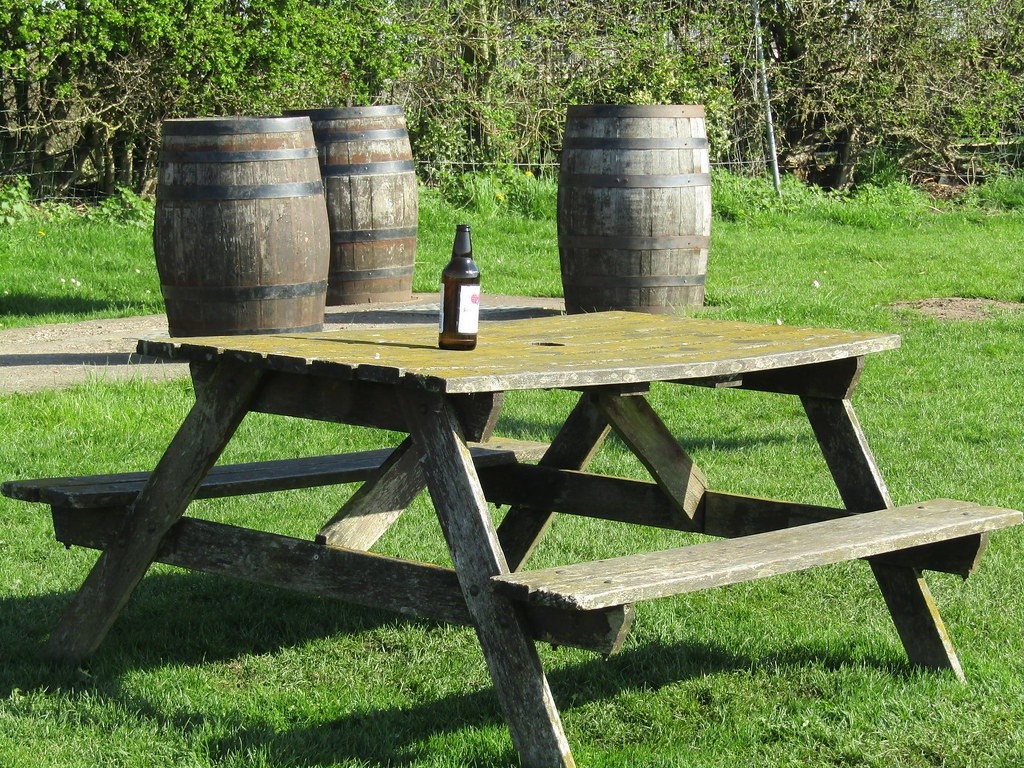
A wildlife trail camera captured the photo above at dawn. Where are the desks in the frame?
[41,311,967,768]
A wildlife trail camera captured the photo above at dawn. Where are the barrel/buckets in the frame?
[156,116,330,337]
[557,105,710,313]
[283,105,417,307]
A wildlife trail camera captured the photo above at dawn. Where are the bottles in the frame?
[438,223,480,351]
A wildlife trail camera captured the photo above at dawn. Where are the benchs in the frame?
[489,499,1023,655]
[0,435,552,548]
